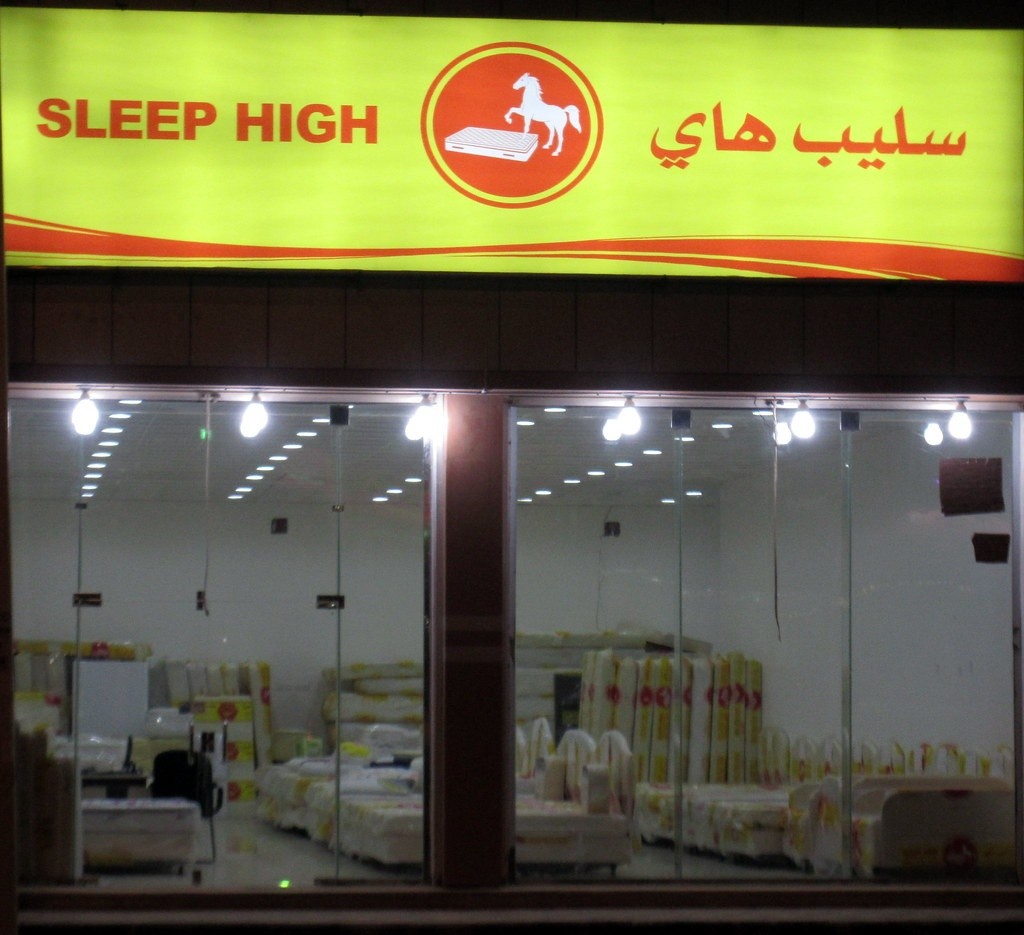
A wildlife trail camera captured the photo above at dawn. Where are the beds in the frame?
[82,797,202,876]
[252,715,1017,882]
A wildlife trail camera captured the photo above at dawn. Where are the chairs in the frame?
[149,749,224,866]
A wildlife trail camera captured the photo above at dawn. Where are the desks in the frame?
[81,771,149,800]
